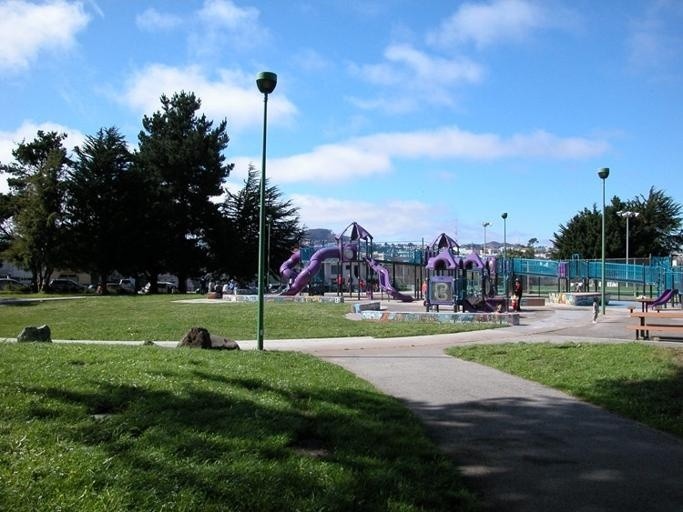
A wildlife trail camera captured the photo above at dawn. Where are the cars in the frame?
[88,278,135,294]
[0,278,29,292]
[49,279,86,294]
[144,281,177,294]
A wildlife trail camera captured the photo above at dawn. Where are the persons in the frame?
[575,282,584,292]
[346,273,353,293]
[198,274,241,296]
[591,296,600,324]
[511,277,522,312]
[510,289,520,312]
[359,279,365,296]
[334,272,345,297]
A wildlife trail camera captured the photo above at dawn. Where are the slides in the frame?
[647,289,678,308]
[363,257,413,303]
[279,248,340,295]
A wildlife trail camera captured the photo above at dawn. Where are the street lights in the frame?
[481,221,489,256]
[616,210,640,288]
